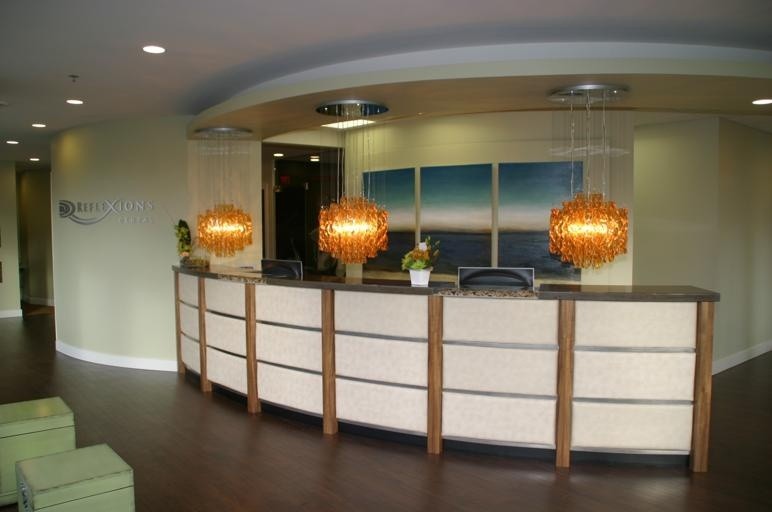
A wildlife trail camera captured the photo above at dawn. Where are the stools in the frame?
[0,395,136,511]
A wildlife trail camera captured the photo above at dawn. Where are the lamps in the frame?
[191,99,392,266]
[547,80,630,272]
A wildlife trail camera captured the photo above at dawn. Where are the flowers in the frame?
[171,218,192,258]
[399,234,442,275]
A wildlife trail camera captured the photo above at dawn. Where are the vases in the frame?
[407,267,431,289]
